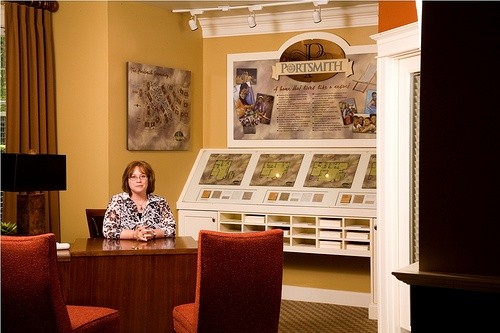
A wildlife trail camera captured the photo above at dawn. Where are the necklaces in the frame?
[132,196,146,210]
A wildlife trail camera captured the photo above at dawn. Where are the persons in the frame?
[236,72,267,124]
[103,161,176,242]
[339,92,376,133]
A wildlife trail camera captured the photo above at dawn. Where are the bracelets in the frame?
[152,229,156,240]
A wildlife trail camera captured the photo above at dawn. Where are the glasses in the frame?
[126,173,148,181]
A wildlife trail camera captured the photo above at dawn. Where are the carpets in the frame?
[278,298,378,333]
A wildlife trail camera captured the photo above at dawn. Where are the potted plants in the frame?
[0,220,18,235]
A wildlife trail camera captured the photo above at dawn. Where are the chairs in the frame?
[86,208,106,238]
[0,233,120,333]
[173,228,284,333]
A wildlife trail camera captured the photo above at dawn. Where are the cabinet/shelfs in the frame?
[176,207,377,258]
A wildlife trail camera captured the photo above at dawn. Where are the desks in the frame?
[56,235,198,333]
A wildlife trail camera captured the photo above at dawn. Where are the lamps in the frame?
[248,11,257,28]
[1,148,67,235]
[188,16,198,31]
[313,6,321,24]
